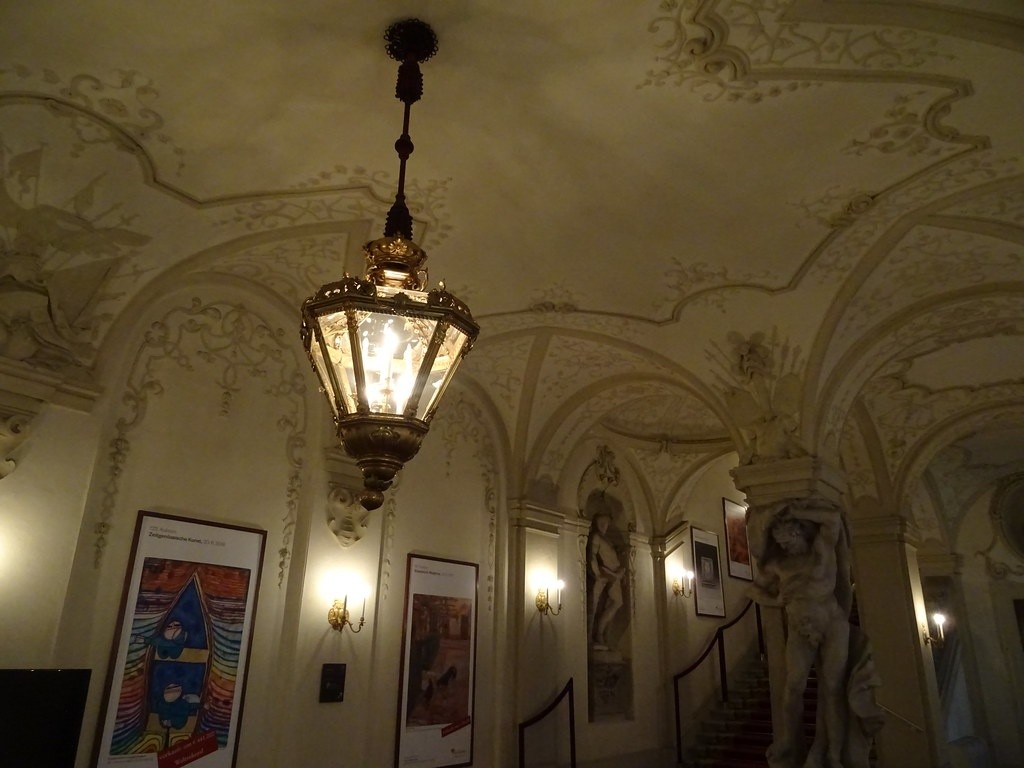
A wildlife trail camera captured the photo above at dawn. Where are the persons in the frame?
[752,498,854,768]
[587,512,627,649]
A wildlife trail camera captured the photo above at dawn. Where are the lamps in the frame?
[328,595,365,632]
[672,576,692,598]
[298,18,481,510]
[923,614,945,647]
[535,586,562,616]
